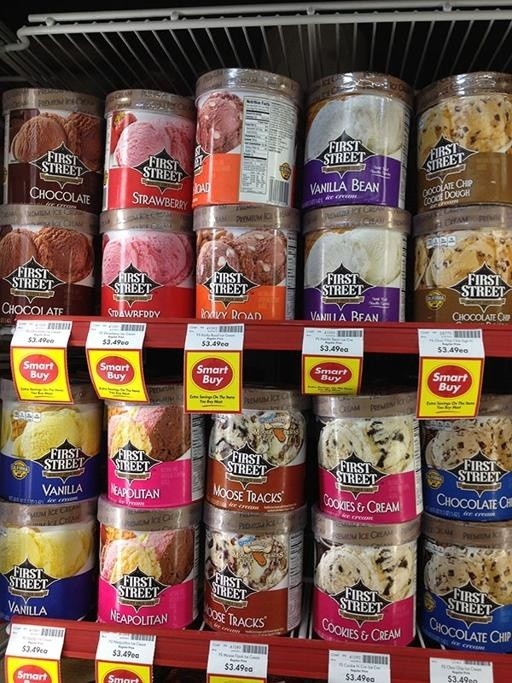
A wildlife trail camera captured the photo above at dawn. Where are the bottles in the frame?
[1,370,104,503]
[310,504,422,645]
[99,491,202,630]
[193,69,299,208]
[413,206,512,324]
[105,385,209,510]
[318,393,427,522]
[0,498,99,622]
[202,498,309,637]
[212,388,310,511]
[413,72,512,212]
[302,205,412,321]
[103,89,194,212]
[420,511,512,655]
[305,72,408,210]
[193,203,299,322]
[424,394,504,524]
[0,204,100,316]
[98,210,194,317]
[0,87,103,212]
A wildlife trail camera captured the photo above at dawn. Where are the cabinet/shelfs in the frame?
[0,315,512,683]
